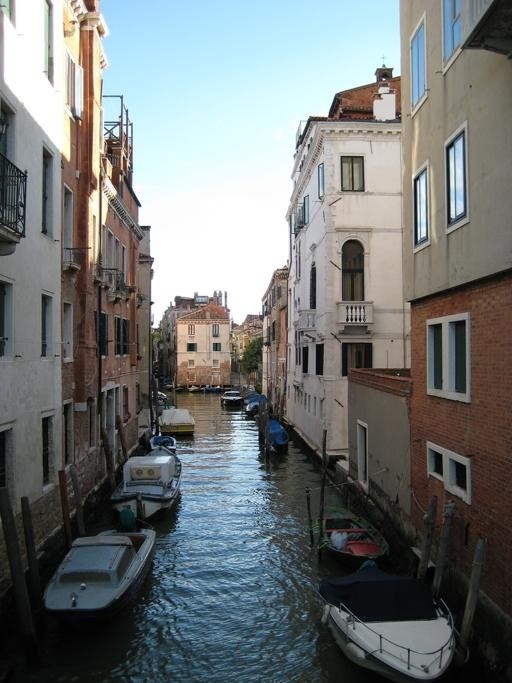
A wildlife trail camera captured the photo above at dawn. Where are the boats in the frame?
[158,409,195,434]
[43,436,182,610]
[220,391,244,406]
[186,384,231,392]
[316,495,457,683]
